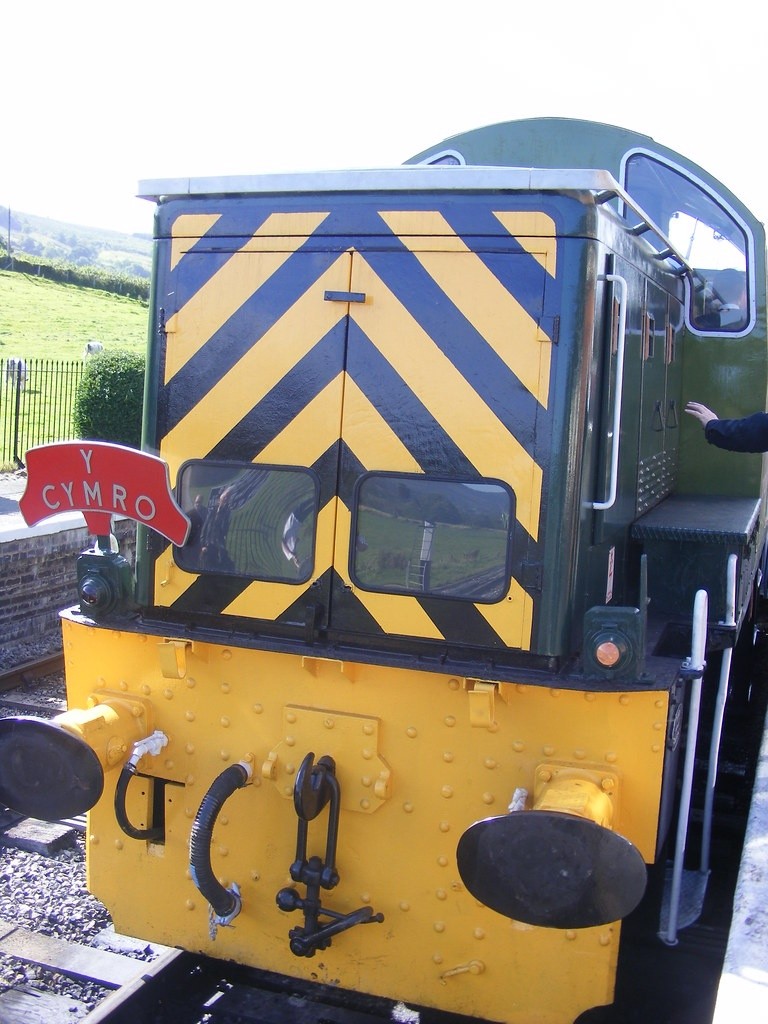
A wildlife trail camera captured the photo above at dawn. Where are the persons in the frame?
[355,530,368,553]
[280,496,315,568]
[199,484,237,571]
[684,401,768,454]
[185,494,208,569]
